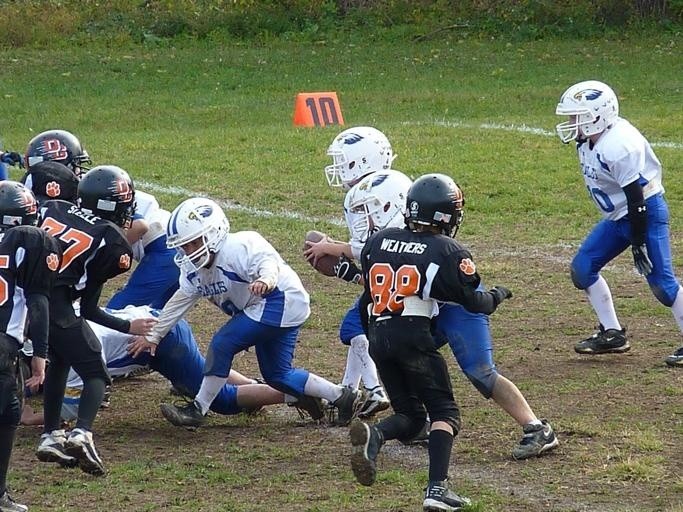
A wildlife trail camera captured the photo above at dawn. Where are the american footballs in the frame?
[305,230,340,275]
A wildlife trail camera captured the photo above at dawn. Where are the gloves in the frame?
[334,252,363,285]
[490,286,513,305]
[631,242,654,277]
[0,150,28,171]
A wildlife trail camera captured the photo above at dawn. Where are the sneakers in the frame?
[35,429,77,468]
[349,422,385,487]
[66,427,106,477]
[159,400,208,432]
[573,324,631,355]
[328,385,363,427]
[294,391,325,422]
[664,346,683,368]
[511,419,559,461]
[423,479,472,512]
[0,490,29,512]
[357,386,390,421]
[402,418,437,447]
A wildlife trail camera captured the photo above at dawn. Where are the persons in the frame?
[556,80,683,368]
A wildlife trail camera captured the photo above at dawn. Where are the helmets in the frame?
[23,129,83,173]
[75,165,135,229]
[0,179,39,228]
[555,78,620,139]
[164,195,231,275]
[401,173,464,237]
[326,125,392,183]
